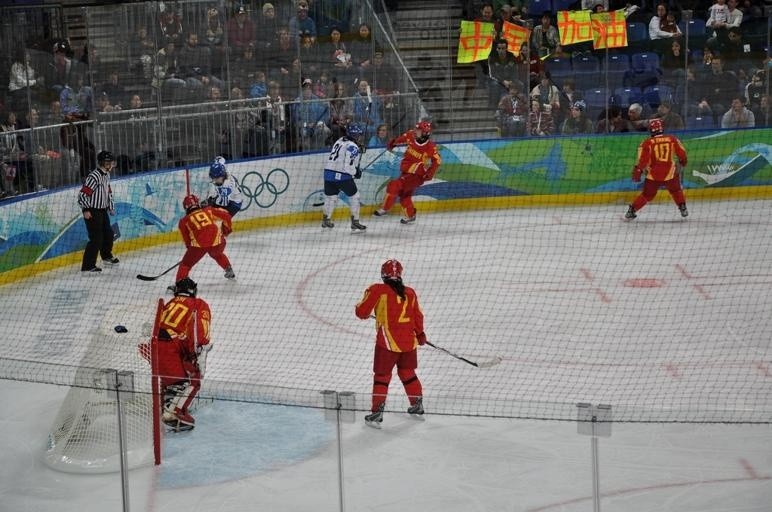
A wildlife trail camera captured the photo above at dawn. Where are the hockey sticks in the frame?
[312,136,399,207]
[369,315,503,369]
[136,262,180,280]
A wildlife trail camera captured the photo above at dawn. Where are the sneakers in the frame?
[365,403,424,424]
[322,208,416,230]
[224,268,235,278]
[625,209,688,219]
[80,256,121,273]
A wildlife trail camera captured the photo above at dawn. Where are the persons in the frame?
[479,2,772,136]
[78,149,117,274]
[318,122,368,230]
[622,119,691,222]
[173,194,236,287]
[1,0,405,202]
[148,276,211,430]
[372,121,440,223]
[355,258,427,423]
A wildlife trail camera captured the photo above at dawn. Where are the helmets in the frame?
[98,151,226,211]
[345,121,433,146]
[381,260,403,282]
[174,278,197,297]
[648,118,665,136]
